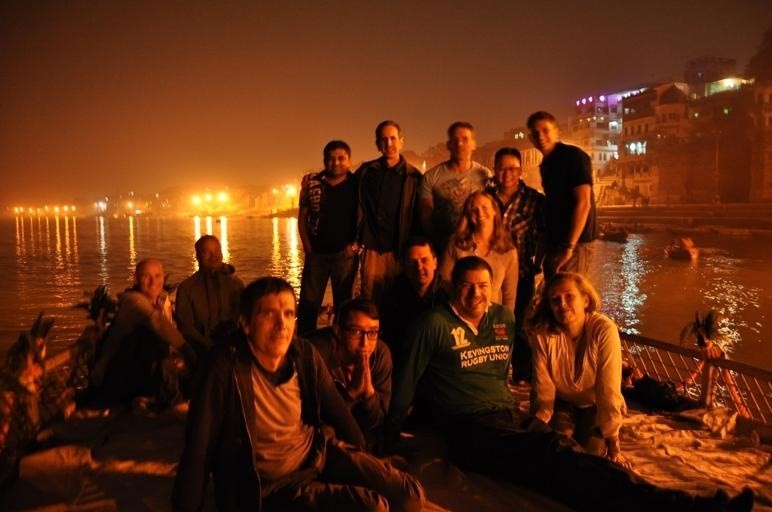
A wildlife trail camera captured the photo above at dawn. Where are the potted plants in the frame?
[679,310,743,362]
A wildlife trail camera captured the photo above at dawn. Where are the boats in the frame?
[597,223,629,242]
[663,240,700,261]
[1,278,772,512]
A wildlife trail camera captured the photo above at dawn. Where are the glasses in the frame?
[346,326,380,340]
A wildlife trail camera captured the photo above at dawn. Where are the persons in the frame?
[109,110,627,512]
